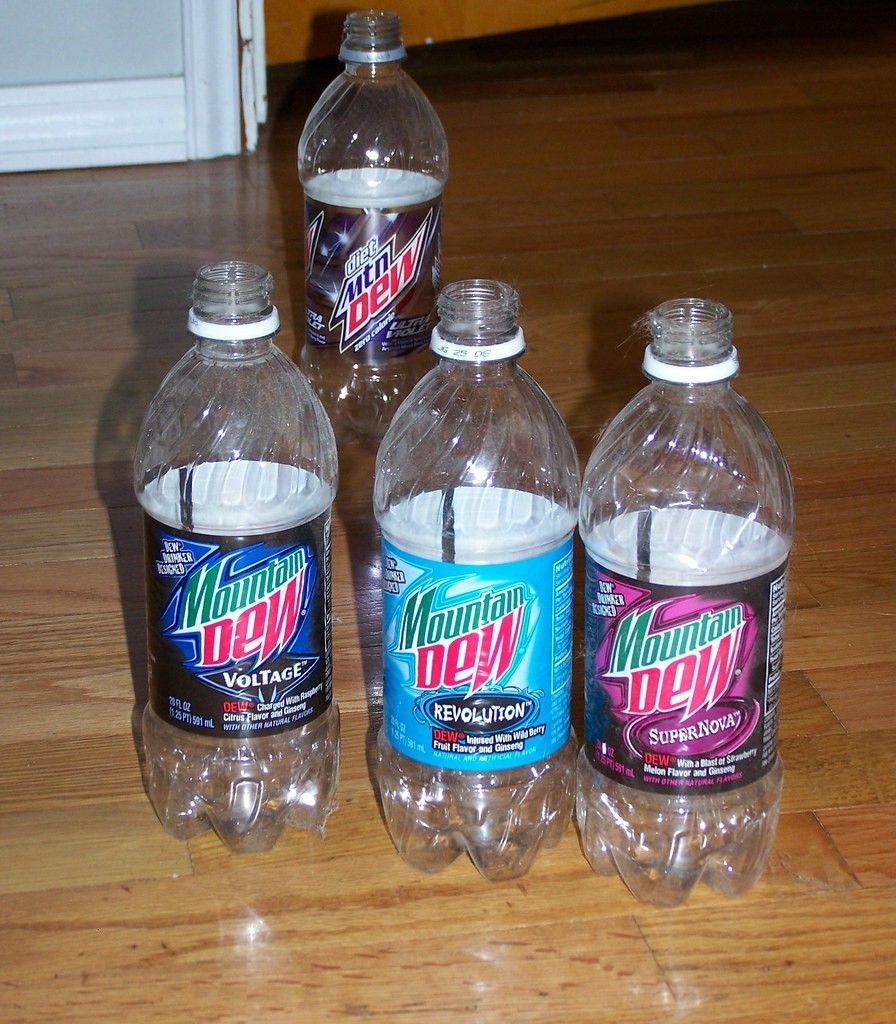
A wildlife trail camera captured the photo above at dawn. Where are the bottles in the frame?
[135,261,343,855]
[579,300,795,908]
[374,278,581,881]
[299,9,450,453]
